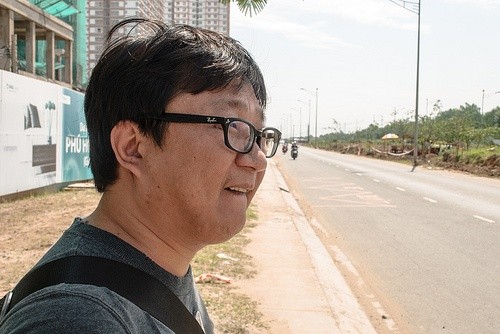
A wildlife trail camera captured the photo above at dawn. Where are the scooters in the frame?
[282,145,288,153]
[290,147,298,160]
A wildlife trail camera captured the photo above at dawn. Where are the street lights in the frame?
[297,98,312,143]
[299,87,318,149]
[482,89,500,116]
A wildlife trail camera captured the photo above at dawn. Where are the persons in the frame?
[0,17,282,334]
[282,142,288,150]
[391,138,404,153]
[291,141,298,154]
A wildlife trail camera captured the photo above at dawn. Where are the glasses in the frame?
[138,112,281,157]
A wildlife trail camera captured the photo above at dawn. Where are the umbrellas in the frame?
[381,133,399,140]
